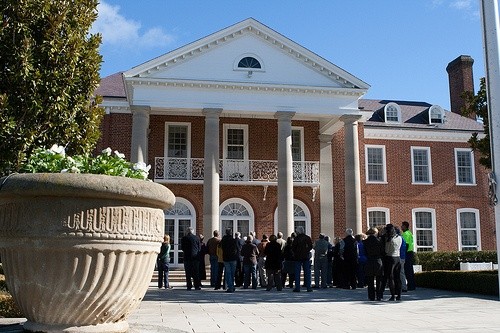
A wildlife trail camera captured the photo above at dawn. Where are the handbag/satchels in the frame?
[217,245,223,261]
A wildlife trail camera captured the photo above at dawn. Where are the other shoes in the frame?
[408,285,416,290]
[389,296,394,300]
[187,287,191,289]
[402,290,407,292]
[377,298,381,300]
[195,288,201,290]
[211,284,367,293]
[396,296,400,300]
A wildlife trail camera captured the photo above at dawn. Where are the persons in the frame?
[157,234,172,290]
[181,221,416,301]
